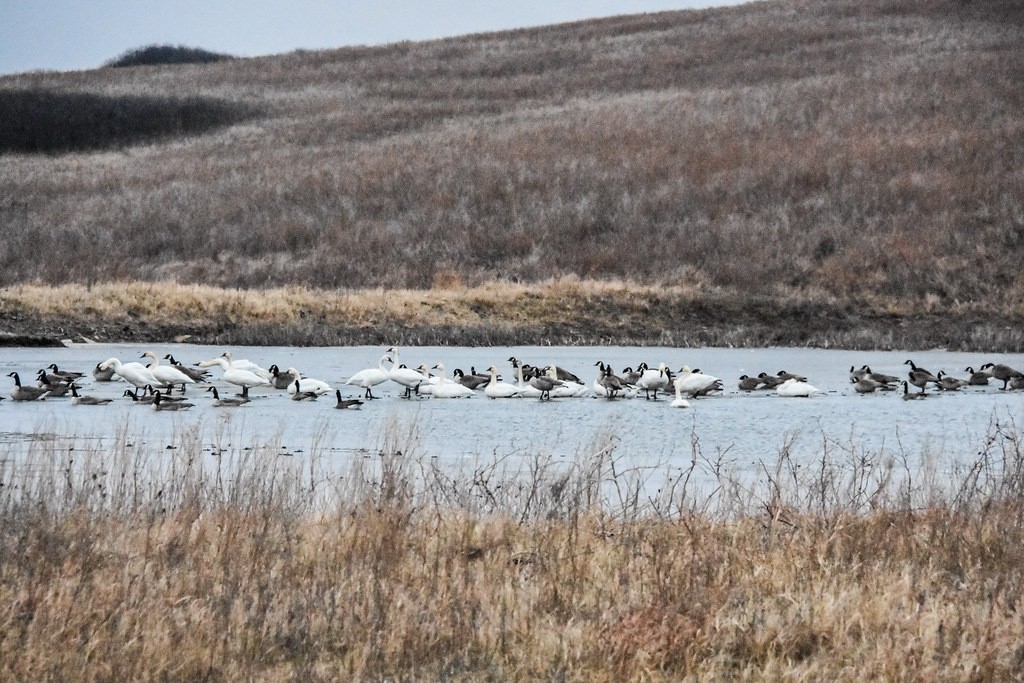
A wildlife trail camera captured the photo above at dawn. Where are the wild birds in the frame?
[849,359,1024,401]
[0,342,822,411]
